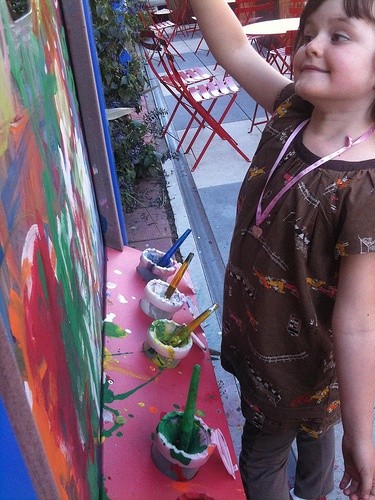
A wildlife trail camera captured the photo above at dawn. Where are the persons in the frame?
[191,0,375,500]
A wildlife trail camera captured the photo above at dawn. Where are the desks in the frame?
[242,17,301,50]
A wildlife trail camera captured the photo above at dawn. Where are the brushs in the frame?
[181,362,201,451]
[159,228,192,266]
[165,252,195,298]
[169,303,218,347]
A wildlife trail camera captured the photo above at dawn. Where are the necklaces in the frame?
[253,119,375,239]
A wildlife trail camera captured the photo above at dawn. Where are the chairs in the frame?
[133,26,228,150]
[166,0,197,37]
[235,2,275,26]
[137,1,187,67]
[158,35,254,170]
[248,28,300,135]
